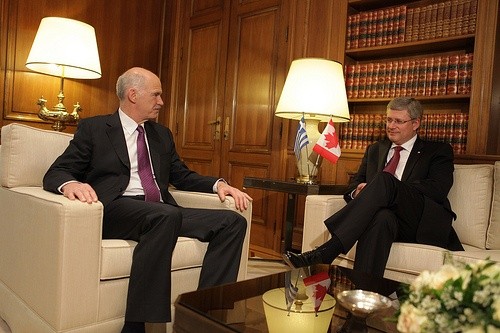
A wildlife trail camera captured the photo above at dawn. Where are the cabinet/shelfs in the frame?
[334,0,489,165]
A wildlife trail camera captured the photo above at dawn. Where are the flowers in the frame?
[381,250,500,333]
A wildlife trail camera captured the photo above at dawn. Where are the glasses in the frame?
[382,118,417,125]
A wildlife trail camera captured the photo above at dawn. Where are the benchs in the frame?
[301,161,500,286]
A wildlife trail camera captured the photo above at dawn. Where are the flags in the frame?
[294,116,309,160]
[284,271,299,315]
[303,270,332,316]
[313,118,342,164]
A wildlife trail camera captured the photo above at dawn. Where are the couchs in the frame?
[0,122,254,333]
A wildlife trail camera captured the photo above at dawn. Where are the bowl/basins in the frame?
[336,289,392,318]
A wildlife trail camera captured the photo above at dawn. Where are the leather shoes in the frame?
[282,247,329,270]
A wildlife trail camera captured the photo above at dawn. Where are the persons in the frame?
[43,66,253,333]
[283,97,465,289]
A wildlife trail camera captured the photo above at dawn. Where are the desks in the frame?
[243,176,348,254]
[169,262,408,333]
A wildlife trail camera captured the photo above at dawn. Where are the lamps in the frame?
[273,56,351,185]
[24,16,103,133]
[262,267,337,333]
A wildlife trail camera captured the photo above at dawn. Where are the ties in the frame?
[136,124,160,202]
[383,145,404,175]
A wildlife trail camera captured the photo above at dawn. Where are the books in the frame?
[340,1,478,155]
